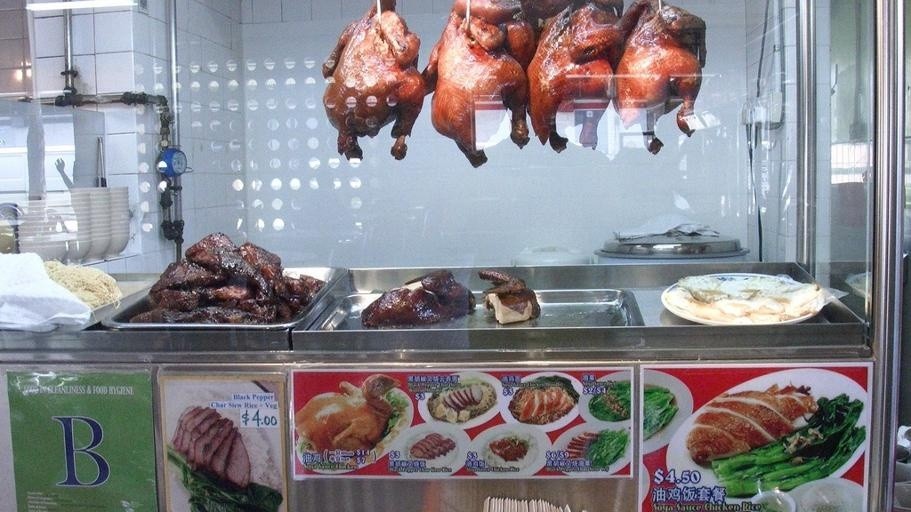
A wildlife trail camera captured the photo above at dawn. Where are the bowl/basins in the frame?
[15,185,131,266]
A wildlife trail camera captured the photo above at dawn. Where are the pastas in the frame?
[44,259,122,310]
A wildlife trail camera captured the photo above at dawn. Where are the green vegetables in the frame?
[711,393,866,496]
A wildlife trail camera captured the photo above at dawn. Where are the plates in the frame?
[659,272,826,326]
[665,366,868,512]
[416,372,503,429]
[389,421,471,479]
[501,371,583,432]
[553,422,632,476]
[466,422,552,480]
[296,386,415,476]
[579,368,692,454]
